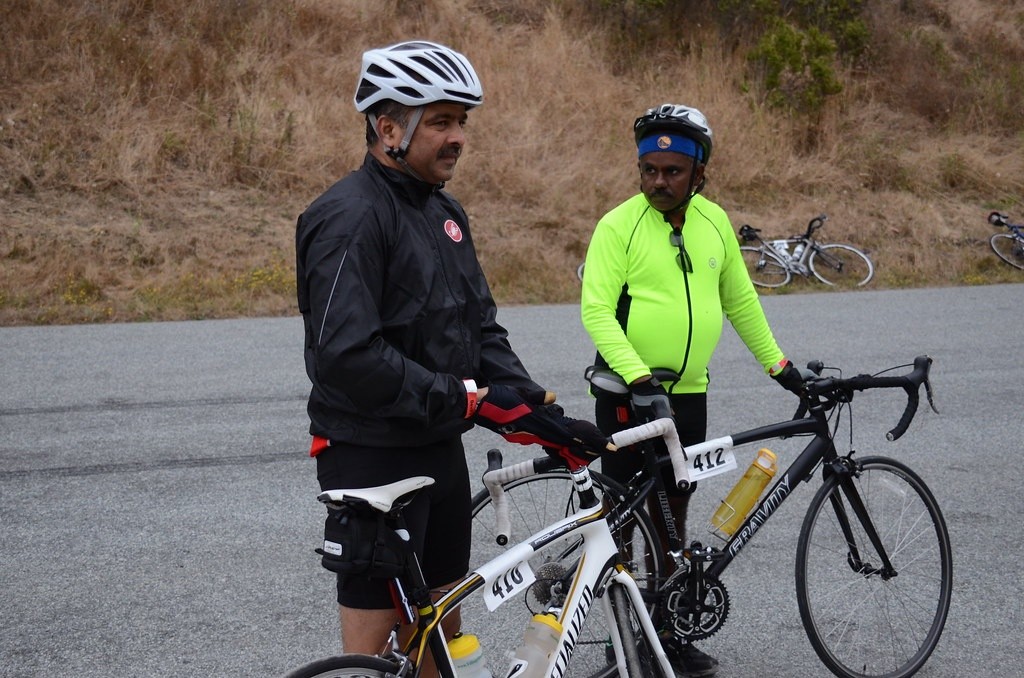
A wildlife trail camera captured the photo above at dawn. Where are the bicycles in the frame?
[459,354,953,678]
[986,212,1024,272]
[739,214,873,288]
[277,418,691,678]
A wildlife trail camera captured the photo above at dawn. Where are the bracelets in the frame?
[768,359,787,375]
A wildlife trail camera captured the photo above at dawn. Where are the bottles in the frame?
[792,242,805,261]
[508,612,563,678]
[447,632,492,678]
[711,449,777,536]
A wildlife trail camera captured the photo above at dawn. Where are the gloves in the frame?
[628,376,677,426]
[770,363,820,398]
[474,384,618,472]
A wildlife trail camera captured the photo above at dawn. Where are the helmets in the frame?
[353,40,485,114]
[634,104,713,166]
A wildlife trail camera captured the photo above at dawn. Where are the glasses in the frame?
[669,226,693,274]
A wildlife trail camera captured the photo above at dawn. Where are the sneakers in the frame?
[604,632,721,678]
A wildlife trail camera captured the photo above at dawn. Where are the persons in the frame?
[293,39,615,678]
[580,106,809,676]
